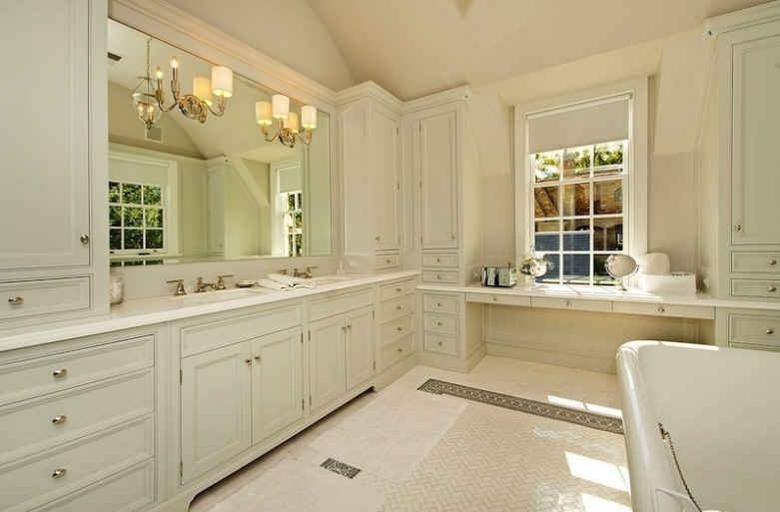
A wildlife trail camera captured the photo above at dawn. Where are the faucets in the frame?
[196,277,214,291]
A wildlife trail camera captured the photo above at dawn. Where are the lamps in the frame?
[129,36,212,140]
[255,101,299,149]
[168,54,236,123]
[272,93,318,145]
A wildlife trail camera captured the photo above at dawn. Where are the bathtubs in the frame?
[613,341,779,512]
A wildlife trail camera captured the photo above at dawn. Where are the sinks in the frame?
[170,289,265,301]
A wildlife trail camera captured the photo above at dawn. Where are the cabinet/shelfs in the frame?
[0,2,107,335]
[1,322,174,512]
[708,0,780,351]
[463,286,714,320]
[176,290,374,488]
[335,99,466,270]
[375,284,486,372]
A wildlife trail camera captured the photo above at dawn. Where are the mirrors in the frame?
[604,254,639,293]
[105,16,331,261]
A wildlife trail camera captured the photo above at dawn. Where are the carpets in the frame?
[202,385,468,512]
